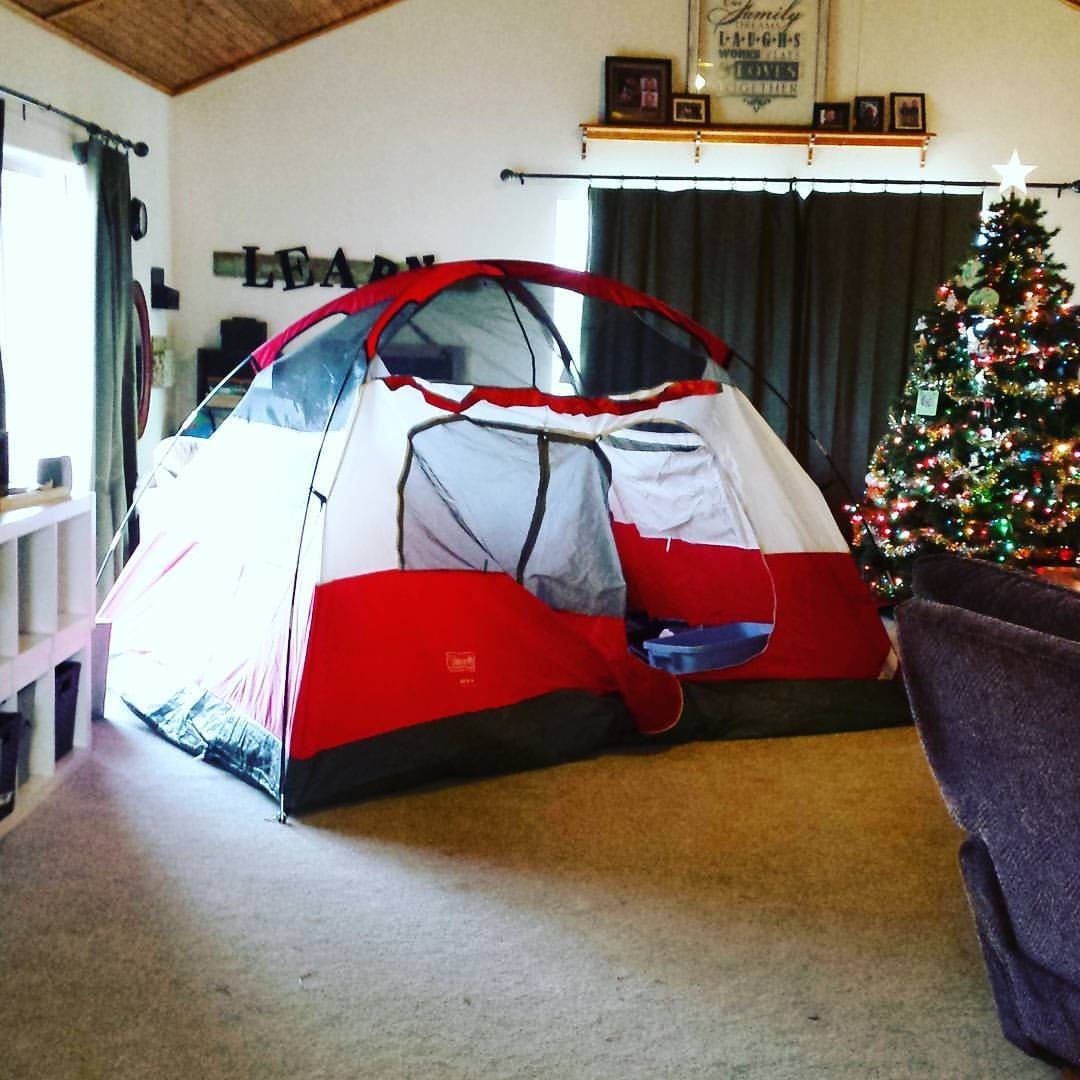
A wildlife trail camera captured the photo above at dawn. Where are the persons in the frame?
[619,76,703,121]
[824,99,919,130]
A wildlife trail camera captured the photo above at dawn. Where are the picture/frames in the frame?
[854,96,884,132]
[671,94,710,128]
[813,102,850,130]
[605,56,672,127]
[890,92,926,133]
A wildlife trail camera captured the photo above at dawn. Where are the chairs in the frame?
[894,554,1080,1070]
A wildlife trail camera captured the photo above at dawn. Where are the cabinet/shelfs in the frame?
[0,490,92,840]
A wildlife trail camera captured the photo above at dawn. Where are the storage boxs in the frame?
[643,622,773,673]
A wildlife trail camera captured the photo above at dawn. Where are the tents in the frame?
[94,258,914,824]
